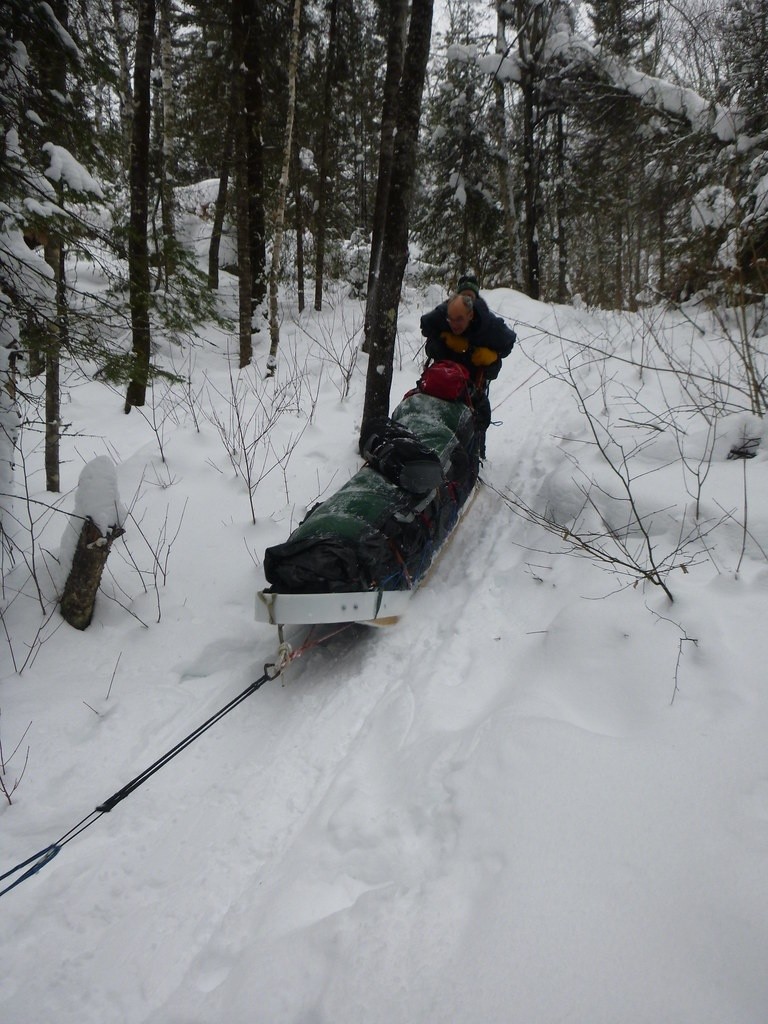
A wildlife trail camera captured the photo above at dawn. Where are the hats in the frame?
[457,276,480,295]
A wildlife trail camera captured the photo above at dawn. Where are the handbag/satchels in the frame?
[417,358,470,400]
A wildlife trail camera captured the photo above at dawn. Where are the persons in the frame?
[421,275,517,358]
[425,295,502,434]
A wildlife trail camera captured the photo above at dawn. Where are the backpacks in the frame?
[359,415,446,497]
